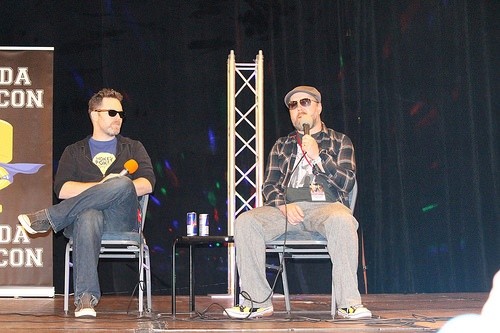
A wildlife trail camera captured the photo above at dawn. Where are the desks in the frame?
[172,235,239,317]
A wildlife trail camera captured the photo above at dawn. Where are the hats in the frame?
[284,85,321,107]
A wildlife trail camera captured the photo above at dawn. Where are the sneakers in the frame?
[17,209,51,234]
[74,293,96,317]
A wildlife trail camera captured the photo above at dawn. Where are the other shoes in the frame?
[223,304,273,317]
[338,304,372,317]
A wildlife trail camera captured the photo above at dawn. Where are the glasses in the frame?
[288,97,318,109]
[95,109,126,117]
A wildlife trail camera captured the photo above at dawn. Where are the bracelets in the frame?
[312,156,321,165]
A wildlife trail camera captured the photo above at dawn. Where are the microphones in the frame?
[300,114,313,135]
[119,159,139,176]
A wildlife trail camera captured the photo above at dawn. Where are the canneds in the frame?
[186,212,198,236]
[199,213,209,236]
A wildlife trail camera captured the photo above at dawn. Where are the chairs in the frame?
[63,194,152,318]
[264,177,358,319]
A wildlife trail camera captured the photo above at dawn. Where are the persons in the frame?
[222,86,372,319]
[18,88,157,317]
